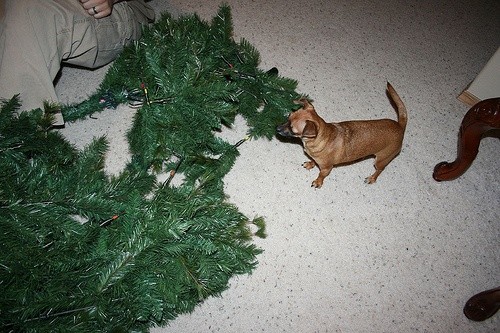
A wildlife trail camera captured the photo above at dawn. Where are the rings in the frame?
[93,7,97,14]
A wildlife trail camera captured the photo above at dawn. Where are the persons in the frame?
[0,0,156,126]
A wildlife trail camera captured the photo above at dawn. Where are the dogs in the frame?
[277,80,408,188]
